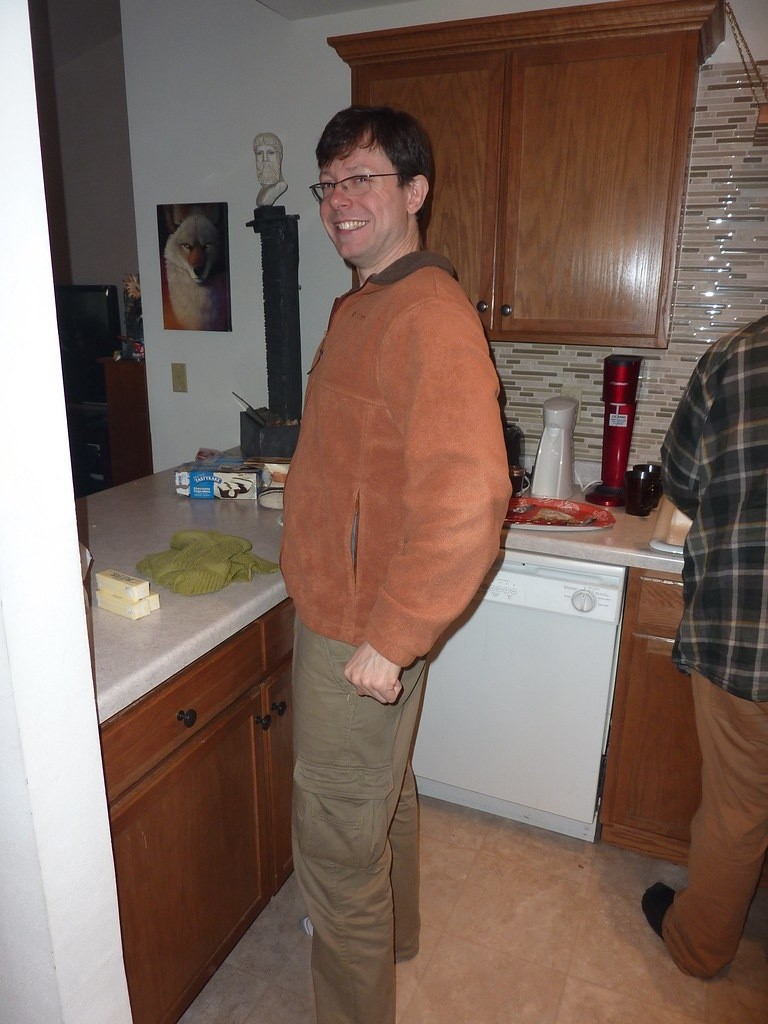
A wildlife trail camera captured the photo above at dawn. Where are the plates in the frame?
[503,496,616,532]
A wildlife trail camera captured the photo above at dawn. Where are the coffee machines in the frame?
[585,354,644,507]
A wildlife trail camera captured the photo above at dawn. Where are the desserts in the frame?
[217,477,253,499]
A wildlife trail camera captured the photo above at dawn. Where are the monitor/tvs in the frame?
[57,285,122,359]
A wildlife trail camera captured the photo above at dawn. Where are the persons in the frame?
[252,132,288,207]
[277,102,514,1022]
[640,317,768,979]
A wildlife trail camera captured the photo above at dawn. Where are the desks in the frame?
[97,563,709,1024]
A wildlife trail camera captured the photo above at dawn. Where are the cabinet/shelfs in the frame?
[305,0,730,355]
[95,354,153,486]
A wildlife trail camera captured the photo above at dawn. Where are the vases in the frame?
[239,412,301,457]
[123,303,144,356]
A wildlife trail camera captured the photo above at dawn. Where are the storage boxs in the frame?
[174,454,291,502]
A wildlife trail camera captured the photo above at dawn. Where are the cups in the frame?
[624,464,664,517]
[508,464,531,499]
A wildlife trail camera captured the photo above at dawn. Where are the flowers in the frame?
[122,271,140,301]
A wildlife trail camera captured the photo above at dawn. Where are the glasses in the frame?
[309,173,400,201]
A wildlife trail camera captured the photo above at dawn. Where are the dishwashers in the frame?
[410,548,629,843]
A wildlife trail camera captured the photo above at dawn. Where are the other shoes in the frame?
[642,881,676,941]
